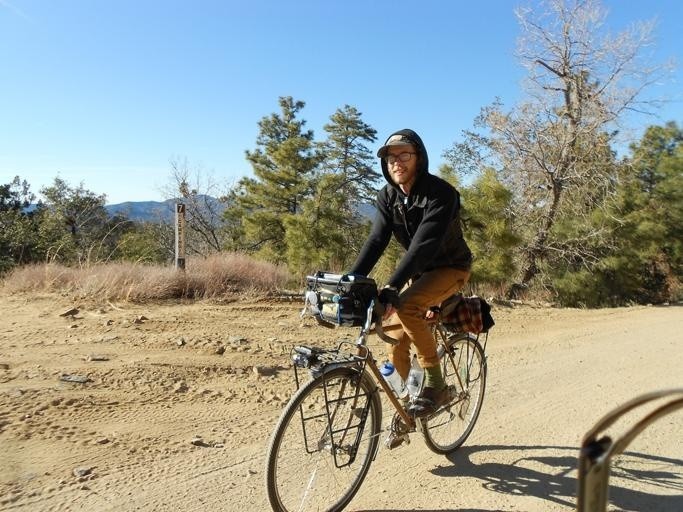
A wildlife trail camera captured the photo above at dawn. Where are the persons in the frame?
[351,128,473,451]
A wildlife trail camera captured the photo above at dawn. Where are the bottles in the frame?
[380,362,410,400]
[404,353,426,399]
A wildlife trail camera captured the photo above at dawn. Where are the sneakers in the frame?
[384,420,408,450]
[407,385,449,417]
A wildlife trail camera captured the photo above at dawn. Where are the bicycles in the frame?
[266,271,487,512]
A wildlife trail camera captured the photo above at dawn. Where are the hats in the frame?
[377,135,416,158]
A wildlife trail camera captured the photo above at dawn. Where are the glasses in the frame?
[384,152,416,163]
